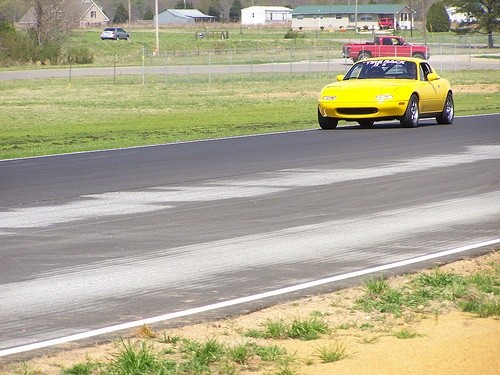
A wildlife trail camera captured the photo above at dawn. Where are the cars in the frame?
[100,27,130,40]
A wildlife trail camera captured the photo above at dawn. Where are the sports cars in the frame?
[316,57,456,130]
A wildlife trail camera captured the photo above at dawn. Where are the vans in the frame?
[378,17,399,29]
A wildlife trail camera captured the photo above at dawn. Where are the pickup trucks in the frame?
[341,35,430,64]
